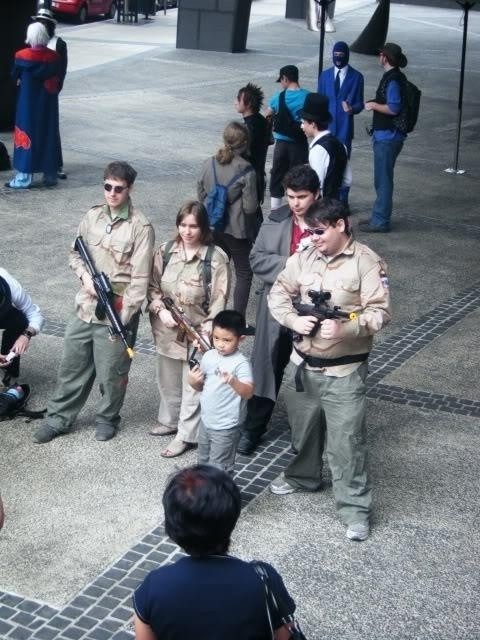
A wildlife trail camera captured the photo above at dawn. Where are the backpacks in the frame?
[204,154,256,235]
[380,71,422,133]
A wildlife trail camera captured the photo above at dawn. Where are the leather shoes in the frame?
[149,424,179,437]
[235,434,261,456]
[160,439,195,458]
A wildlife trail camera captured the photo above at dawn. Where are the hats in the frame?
[30,8,59,25]
[276,65,299,85]
[294,92,334,123]
[379,40,407,68]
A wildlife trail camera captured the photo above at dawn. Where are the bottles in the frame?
[7,385,26,399]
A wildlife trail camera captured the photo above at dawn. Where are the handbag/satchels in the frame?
[273,88,308,143]
[0,381,31,423]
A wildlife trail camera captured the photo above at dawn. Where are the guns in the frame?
[73,234,135,359]
[164,297,211,353]
[188,347,201,370]
[292,290,357,342]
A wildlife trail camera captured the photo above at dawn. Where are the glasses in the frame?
[103,183,128,193]
[303,223,332,235]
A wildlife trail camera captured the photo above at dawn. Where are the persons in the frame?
[358,42,411,232]
[268,200,392,540]
[236,82,270,202]
[32,162,155,443]
[29,8,68,180]
[148,201,231,457]
[318,41,364,215]
[0,268,44,420]
[5,23,64,188]
[188,310,255,475]
[261,65,310,210]
[197,122,263,334]
[296,92,351,201]
[131,463,299,640]
[237,166,320,455]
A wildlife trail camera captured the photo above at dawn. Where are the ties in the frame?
[334,70,342,98]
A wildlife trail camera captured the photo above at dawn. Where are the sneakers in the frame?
[268,480,297,496]
[31,427,62,445]
[4,170,67,187]
[242,324,256,337]
[358,219,391,233]
[346,523,371,542]
[95,424,117,441]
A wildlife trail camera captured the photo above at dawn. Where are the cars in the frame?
[52,0,117,24]
